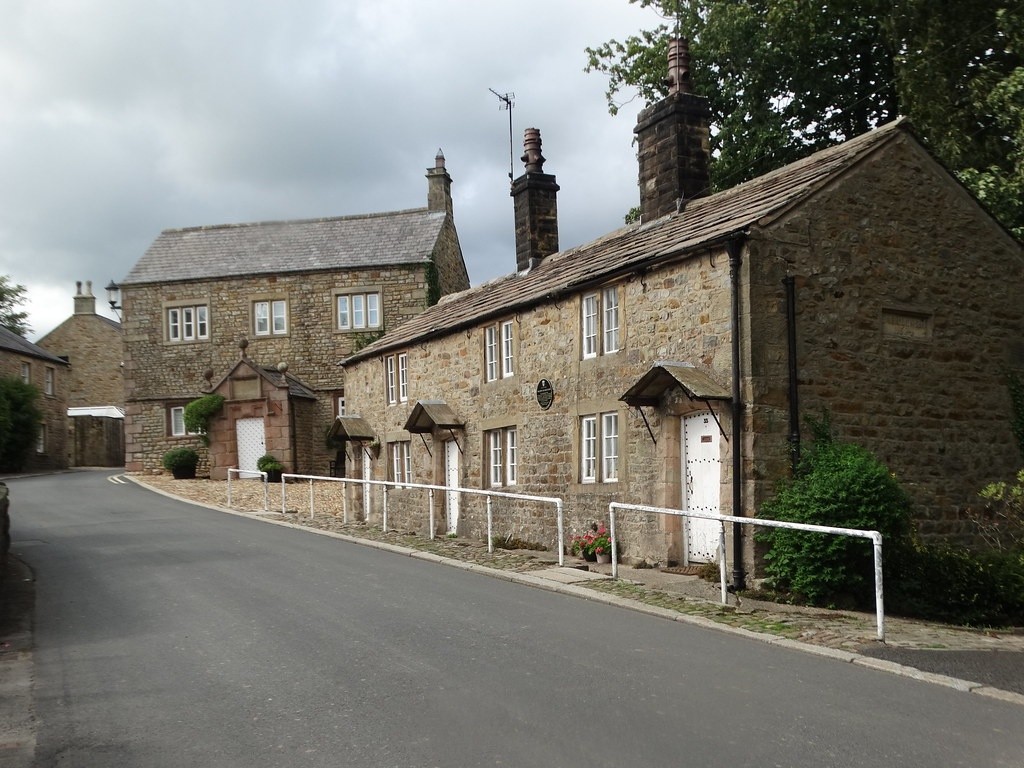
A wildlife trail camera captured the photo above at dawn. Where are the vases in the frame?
[582,549,597,562]
[595,550,609,563]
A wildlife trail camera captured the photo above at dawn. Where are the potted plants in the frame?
[256,455,285,482]
[162,446,199,479]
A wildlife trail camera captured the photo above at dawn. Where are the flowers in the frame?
[571,522,620,555]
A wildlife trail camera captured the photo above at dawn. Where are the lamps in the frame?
[105,279,122,323]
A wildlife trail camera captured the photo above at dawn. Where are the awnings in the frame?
[67,406,125,421]
[327,415,377,462]
[618,366,733,444]
[403,400,465,458]
